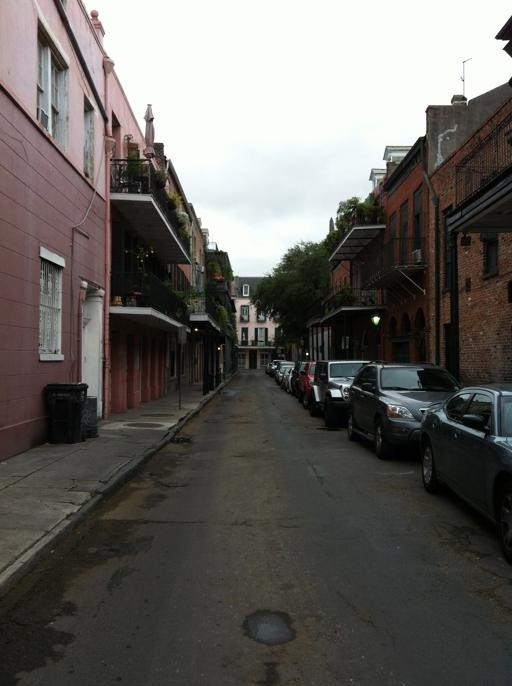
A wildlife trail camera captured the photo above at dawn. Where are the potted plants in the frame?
[154,169,167,189]
[167,192,179,212]
[176,212,189,228]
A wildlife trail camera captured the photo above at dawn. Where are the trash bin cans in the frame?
[46,383,88,444]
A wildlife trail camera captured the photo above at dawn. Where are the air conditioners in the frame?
[412,248,425,265]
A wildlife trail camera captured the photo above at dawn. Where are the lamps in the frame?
[460,233,471,247]
[371,313,381,326]
